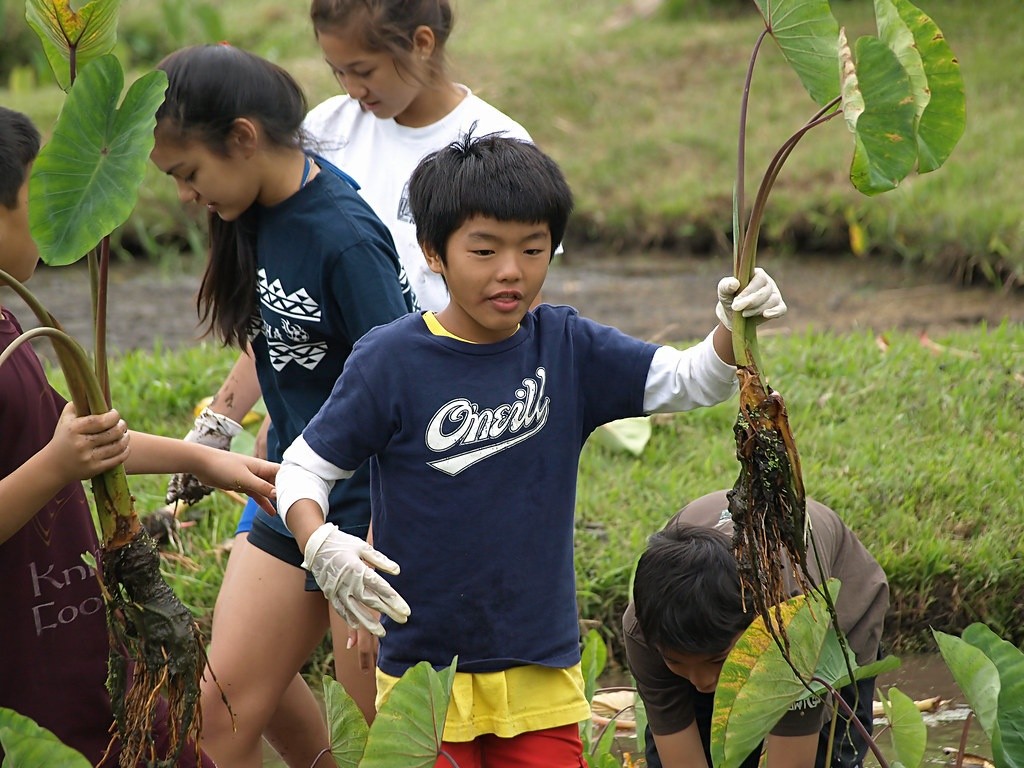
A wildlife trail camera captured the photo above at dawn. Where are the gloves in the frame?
[166,406,244,507]
[715,267,788,332]
[300,522,411,638]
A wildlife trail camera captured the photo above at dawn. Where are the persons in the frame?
[149,42,423,768]
[621,487,888,768]
[0,107,283,768]
[227,0,567,768]
[276,120,789,768]
